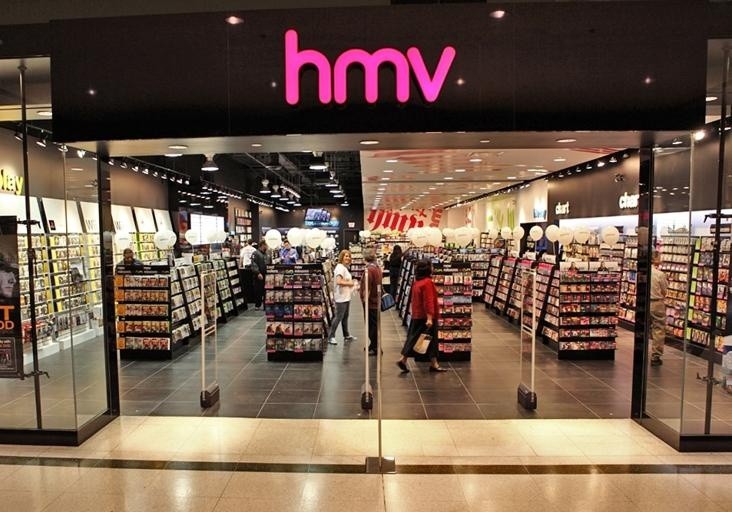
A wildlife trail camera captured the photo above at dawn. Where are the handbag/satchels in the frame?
[381,293,395,311]
[412,334,433,355]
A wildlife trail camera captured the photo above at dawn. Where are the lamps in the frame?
[506,134,684,193]
[12,124,352,211]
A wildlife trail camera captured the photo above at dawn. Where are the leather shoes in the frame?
[396,360,409,372]
[429,366,448,372]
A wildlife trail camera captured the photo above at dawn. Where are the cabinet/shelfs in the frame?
[17,227,731,364]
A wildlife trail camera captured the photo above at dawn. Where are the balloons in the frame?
[185,229,199,245]
[557,226,573,245]
[602,226,619,247]
[217,230,226,243]
[207,230,217,242]
[530,226,543,240]
[513,227,525,240]
[265,227,371,249]
[575,225,591,245]
[406,226,480,249]
[164,228,177,247]
[114,230,131,250]
[545,224,558,242]
[154,231,169,250]
[501,226,512,239]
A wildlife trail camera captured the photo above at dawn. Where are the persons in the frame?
[238,235,321,313]
[326,250,359,345]
[494,232,504,248]
[388,244,412,305]
[0,243,19,307]
[598,261,610,271]
[397,258,449,372]
[360,248,384,357]
[649,250,668,366]
[114,248,144,273]
[567,262,581,271]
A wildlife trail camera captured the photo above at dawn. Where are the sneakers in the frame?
[328,337,337,344]
[344,336,357,342]
[364,348,382,356]
